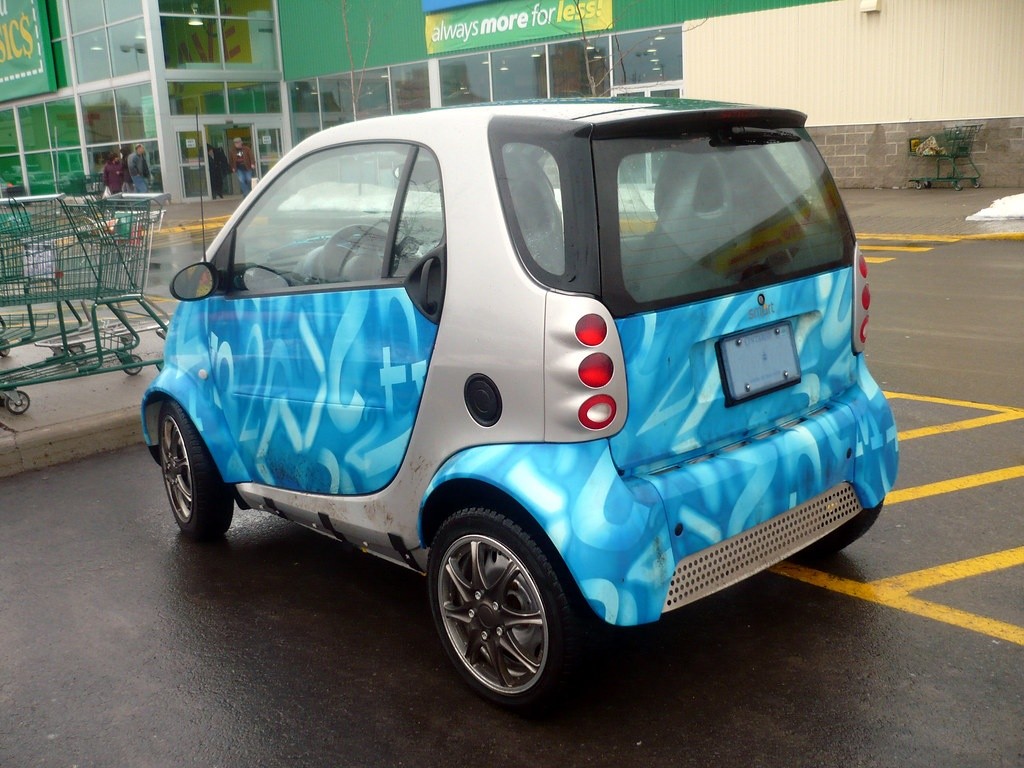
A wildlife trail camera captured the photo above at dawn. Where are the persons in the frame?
[103,144,150,210]
[230,138,255,198]
[207,143,228,199]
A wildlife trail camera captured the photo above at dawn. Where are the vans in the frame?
[136,96,902,711]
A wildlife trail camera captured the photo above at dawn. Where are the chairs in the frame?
[635,150,740,301]
[503,153,565,273]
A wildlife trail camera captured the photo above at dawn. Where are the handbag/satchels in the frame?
[102,186,111,199]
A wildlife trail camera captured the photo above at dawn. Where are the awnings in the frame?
[425,0,839,58]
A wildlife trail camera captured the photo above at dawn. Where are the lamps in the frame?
[499,58,509,71]
[188,0,204,25]
[647,30,666,71]
[530,47,540,57]
[586,40,602,58]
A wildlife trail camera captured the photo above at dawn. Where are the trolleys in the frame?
[0,189,174,416]
[907,123,984,192]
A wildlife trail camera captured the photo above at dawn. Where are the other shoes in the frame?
[213,193,223,200]
